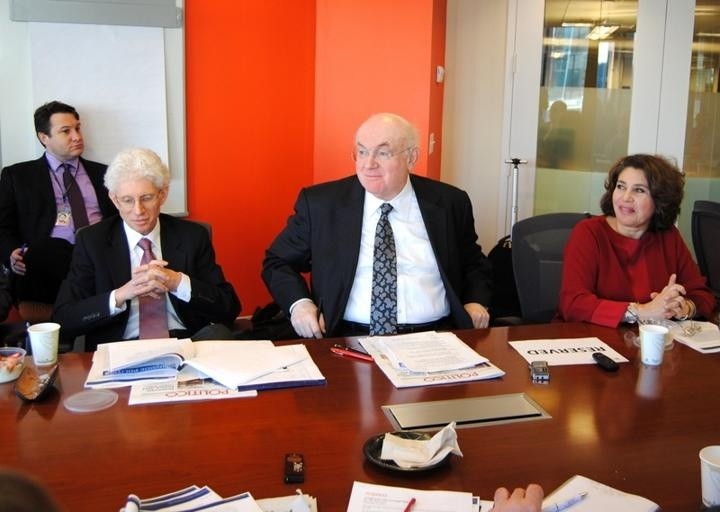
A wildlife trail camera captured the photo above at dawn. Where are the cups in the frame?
[638,325,667,363]
[26,321,61,365]
[698,445,720,509]
[635,363,664,399]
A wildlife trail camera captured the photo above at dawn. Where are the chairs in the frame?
[482,199,720,319]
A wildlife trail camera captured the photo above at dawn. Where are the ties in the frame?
[370,203,397,337]
[138,239,169,340]
[62,164,89,232]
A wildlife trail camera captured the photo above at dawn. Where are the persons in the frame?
[261,112,493,339]
[53,146,242,353]
[0,469,63,511]
[489,482,545,512]
[1,101,122,298]
[537,101,568,165]
[552,153,717,330]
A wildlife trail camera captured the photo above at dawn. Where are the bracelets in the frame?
[625,302,638,324]
[677,299,696,320]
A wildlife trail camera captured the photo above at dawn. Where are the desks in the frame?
[0,318,720,508]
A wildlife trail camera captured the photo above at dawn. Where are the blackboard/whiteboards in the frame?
[0,0,188,220]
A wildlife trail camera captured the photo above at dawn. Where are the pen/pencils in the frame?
[404,498,416,512]
[542,492,589,511]
[329,344,375,362]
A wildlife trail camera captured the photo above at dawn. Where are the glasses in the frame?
[114,190,160,207]
[352,145,414,160]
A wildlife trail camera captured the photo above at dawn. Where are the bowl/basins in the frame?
[0,346,26,383]
[637,318,678,346]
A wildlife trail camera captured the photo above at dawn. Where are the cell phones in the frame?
[285,453,304,482]
[532,361,549,380]
[593,352,618,372]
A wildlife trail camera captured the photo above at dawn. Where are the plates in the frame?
[363,431,452,472]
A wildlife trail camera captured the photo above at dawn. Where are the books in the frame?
[85,336,327,391]
[663,320,720,349]
